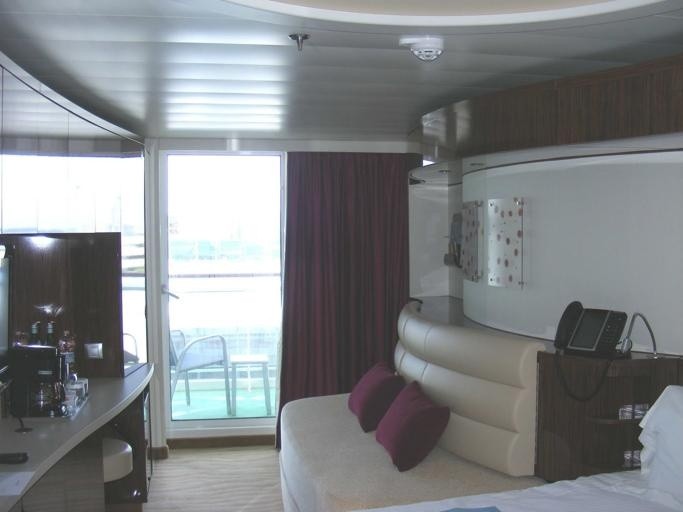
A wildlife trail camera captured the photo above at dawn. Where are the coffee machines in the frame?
[9,345,65,417]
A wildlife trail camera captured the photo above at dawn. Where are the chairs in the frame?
[162,310,230,416]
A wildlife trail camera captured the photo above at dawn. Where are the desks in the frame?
[0,362,157,512]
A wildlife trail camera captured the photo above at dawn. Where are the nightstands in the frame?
[532,340,683,486]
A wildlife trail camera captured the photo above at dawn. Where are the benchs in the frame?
[278,299,547,512]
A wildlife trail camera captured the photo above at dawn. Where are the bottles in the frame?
[61,378,88,416]
[9,322,79,377]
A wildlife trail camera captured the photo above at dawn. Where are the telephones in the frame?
[553,300,628,359]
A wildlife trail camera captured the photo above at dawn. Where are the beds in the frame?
[357,465,683,511]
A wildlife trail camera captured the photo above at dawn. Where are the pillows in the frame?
[638,385,683,504]
[348,357,407,433]
[375,378,451,473]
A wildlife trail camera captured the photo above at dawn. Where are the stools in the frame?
[102,438,142,512]
[224,350,273,418]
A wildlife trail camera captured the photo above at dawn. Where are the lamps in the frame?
[616,310,663,362]
